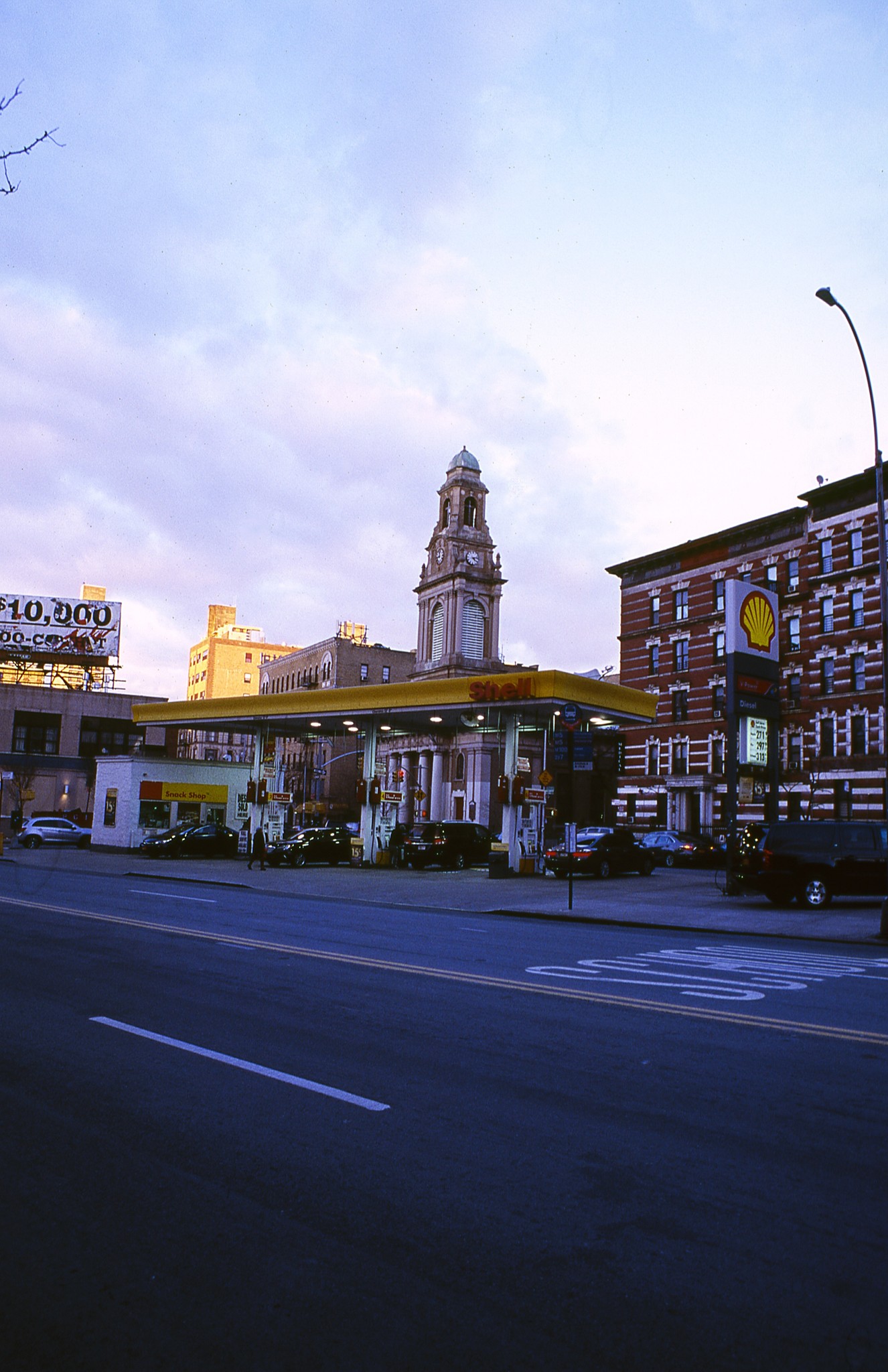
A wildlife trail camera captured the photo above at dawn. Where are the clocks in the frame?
[435,548,444,565]
[466,551,479,565]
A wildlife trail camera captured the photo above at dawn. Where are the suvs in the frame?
[402,818,503,872]
[728,821,888,909]
[17,817,93,850]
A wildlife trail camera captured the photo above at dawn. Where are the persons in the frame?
[387,824,406,868]
[246,826,267,871]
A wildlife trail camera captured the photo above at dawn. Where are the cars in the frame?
[324,819,347,828]
[575,826,721,869]
[139,822,239,860]
[543,833,658,877]
[262,827,361,868]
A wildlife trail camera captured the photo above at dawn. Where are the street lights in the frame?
[816,285,888,822]
